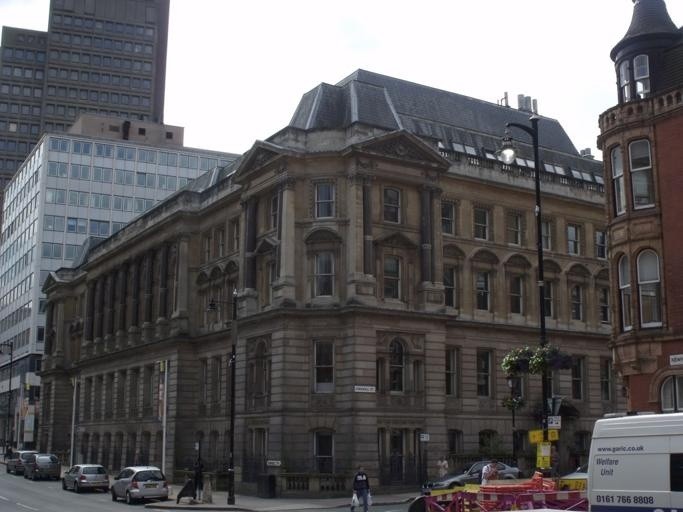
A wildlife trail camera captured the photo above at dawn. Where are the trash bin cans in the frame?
[257,473,276,498]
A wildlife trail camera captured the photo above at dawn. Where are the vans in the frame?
[587,411,683,512]
[6,451,61,481]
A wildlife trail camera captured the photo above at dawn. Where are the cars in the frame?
[561,461,589,479]
[62,464,109,494]
[422,459,522,491]
[111,466,169,505]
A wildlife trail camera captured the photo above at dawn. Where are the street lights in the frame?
[0,340,13,447]
[495,111,557,452]
[70,376,82,469]
[154,359,168,478]
[205,287,238,506]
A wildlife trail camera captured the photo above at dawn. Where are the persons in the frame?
[350,464,370,512]
[193,457,203,491]
[480,458,498,485]
[435,454,448,477]
